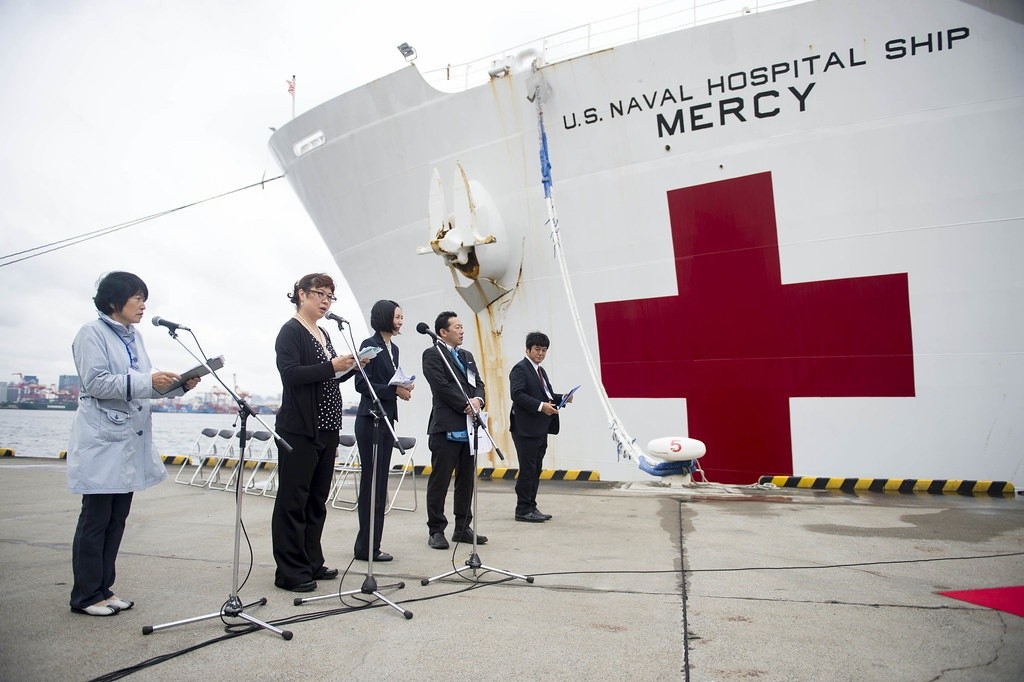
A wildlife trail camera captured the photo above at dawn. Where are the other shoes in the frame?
[452,529,489,544]
[287,580,317,591]
[105,595,134,610]
[532,508,552,518]
[313,568,339,580]
[71,600,121,617]
[515,511,546,522]
[428,533,449,549]
[375,551,393,561]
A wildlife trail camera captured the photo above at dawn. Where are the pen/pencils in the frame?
[155,366,179,383]
[340,354,361,359]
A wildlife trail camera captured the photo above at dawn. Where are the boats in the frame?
[266,0,1024,494]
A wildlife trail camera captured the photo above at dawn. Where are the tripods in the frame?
[142,329,295,641]
[294,321,414,620]
[420,337,535,585]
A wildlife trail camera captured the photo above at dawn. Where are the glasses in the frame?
[309,288,337,303]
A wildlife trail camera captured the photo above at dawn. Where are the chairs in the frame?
[332,448,364,512]
[324,434,356,506]
[176,428,279,499]
[382,437,417,514]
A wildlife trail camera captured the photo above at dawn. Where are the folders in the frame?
[558,385,581,410]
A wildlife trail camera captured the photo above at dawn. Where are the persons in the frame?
[272,272,371,592]
[355,300,414,564]
[69,270,201,617]
[421,311,488,551]
[509,332,573,523]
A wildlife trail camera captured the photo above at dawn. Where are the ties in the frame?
[451,349,466,377]
[538,367,544,386]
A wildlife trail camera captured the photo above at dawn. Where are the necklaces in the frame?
[297,313,327,352]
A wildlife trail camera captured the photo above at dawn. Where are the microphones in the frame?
[417,322,445,343]
[152,316,190,330]
[325,311,349,324]
